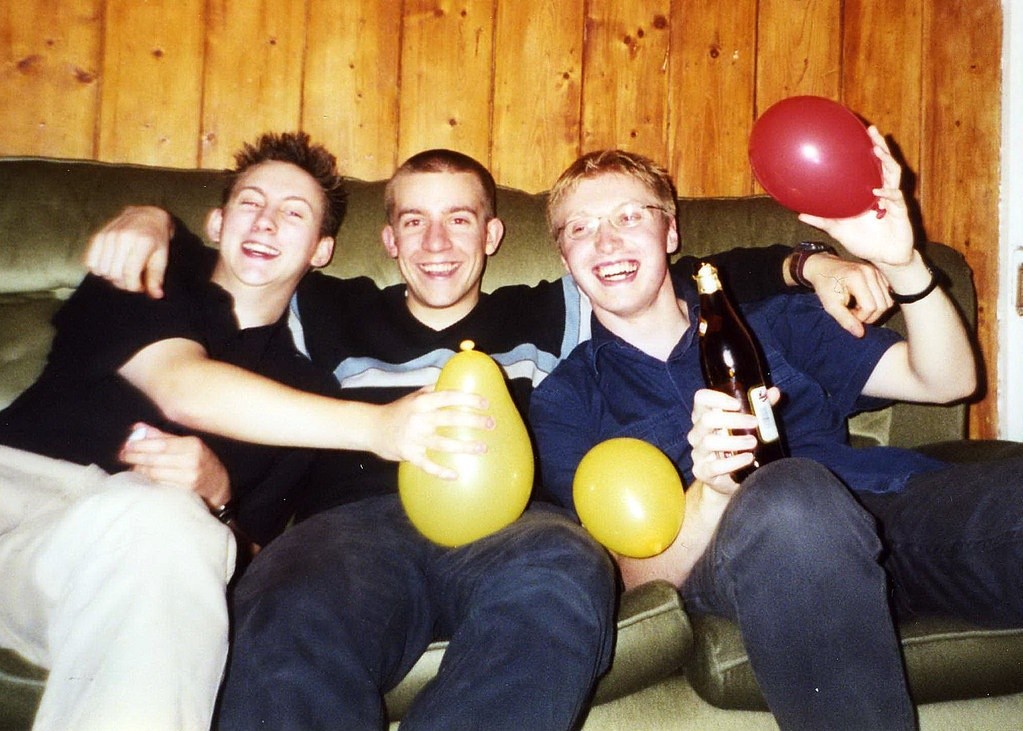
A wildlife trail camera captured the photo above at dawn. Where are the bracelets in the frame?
[888,266,939,305]
[212,498,235,525]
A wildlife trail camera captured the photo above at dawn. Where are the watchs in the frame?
[788,241,837,292]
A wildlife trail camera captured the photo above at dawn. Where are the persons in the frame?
[0,134,496,731]
[85,148,896,731]
[530,126,1023,731]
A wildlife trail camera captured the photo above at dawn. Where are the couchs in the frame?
[0,155,1023,731]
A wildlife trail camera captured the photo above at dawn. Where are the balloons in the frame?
[397,340,534,548]
[572,437,687,557]
[748,96,886,220]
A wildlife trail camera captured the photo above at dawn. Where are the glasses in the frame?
[552,205,667,242]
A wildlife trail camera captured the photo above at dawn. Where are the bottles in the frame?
[693,258,784,485]
